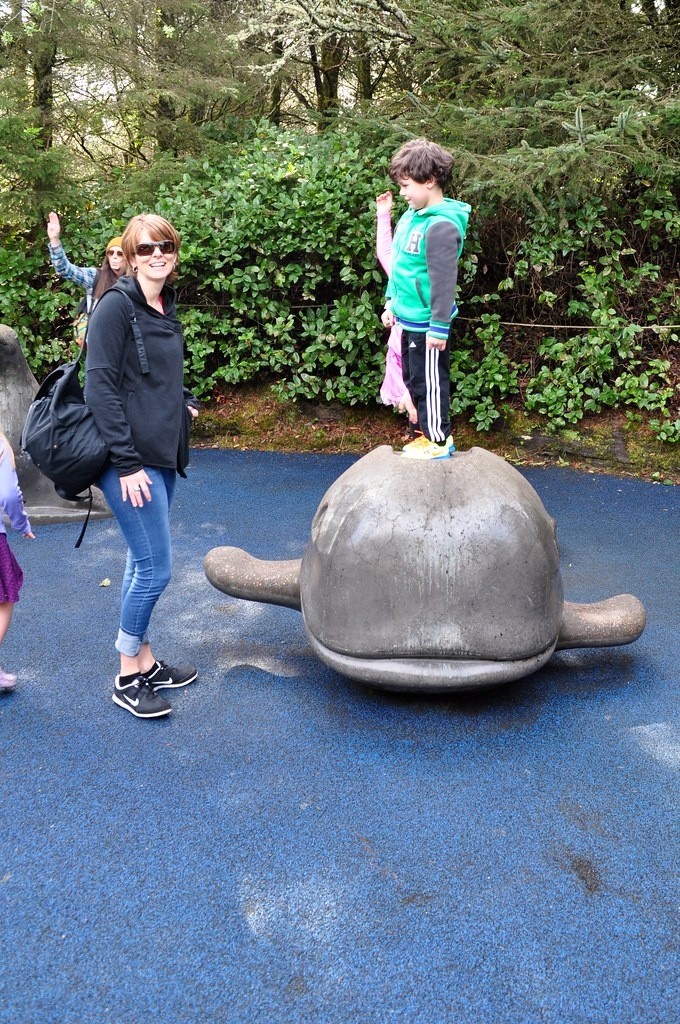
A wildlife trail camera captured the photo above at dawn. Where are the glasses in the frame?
[132,240,178,256]
[107,250,126,256]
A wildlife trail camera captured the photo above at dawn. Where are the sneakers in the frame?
[142,660,199,692]
[402,439,450,459]
[112,674,173,718]
[402,432,455,453]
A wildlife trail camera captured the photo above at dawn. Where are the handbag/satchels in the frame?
[19,287,151,502]
[73,269,102,349]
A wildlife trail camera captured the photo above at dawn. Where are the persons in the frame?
[375,138,472,459]
[86,213,200,718]
[0,210,134,690]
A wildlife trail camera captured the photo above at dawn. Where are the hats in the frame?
[104,236,129,255]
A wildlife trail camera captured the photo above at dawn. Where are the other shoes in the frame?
[0,666,17,692]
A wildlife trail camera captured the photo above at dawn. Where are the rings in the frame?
[135,487,141,491]
